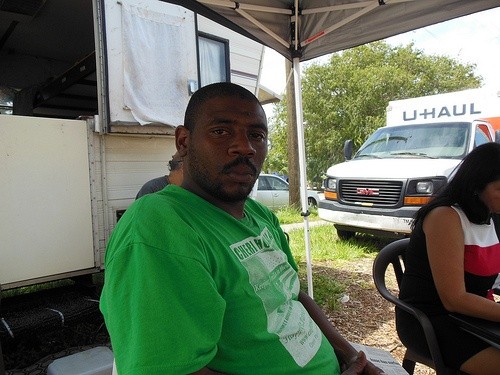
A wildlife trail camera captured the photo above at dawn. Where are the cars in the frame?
[274,173,289,183]
[248,174,325,209]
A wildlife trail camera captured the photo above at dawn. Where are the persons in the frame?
[99,82,385,375]
[135,155,184,200]
[395,142,500,375]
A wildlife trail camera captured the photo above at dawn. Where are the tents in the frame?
[138,0,500,298]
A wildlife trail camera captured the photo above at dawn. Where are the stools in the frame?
[47,346,114,375]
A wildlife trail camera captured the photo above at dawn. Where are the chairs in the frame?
[373,238,500,375]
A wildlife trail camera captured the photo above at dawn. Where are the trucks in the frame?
[318,86,499,242]
